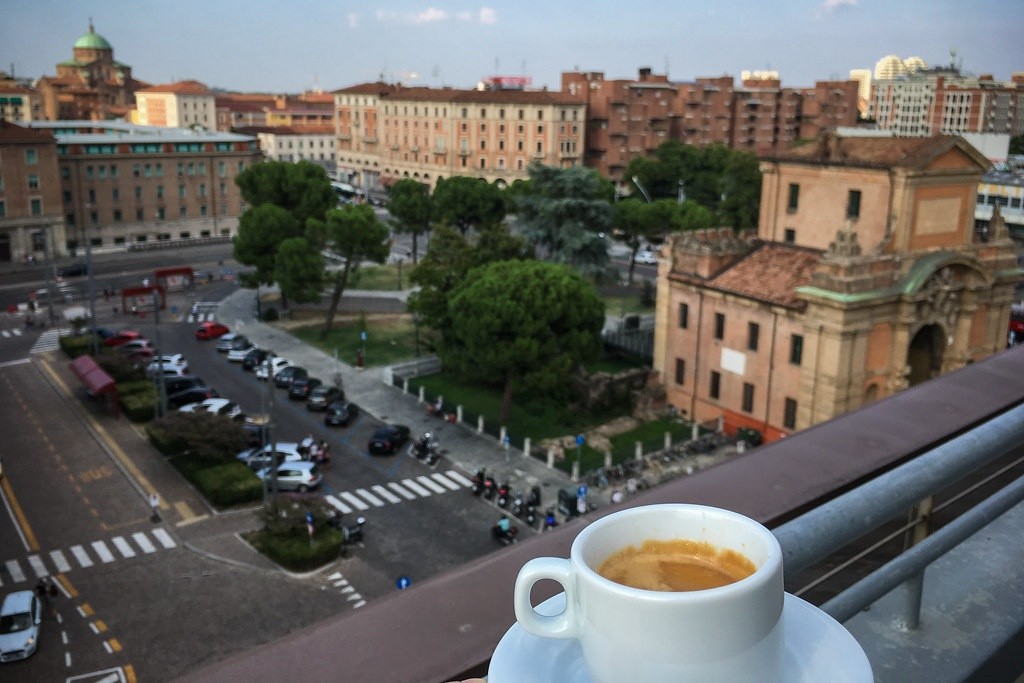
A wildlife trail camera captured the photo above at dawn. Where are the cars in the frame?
[289,377,323,400]
[256,356,297,380]
[216,332,249,353]
[274,366,307,388]
[100,331,242,419]
[633,251,658,265]
[306,384,341,411]
[227,343,258,362]
[327,399,357,427]
[368,425,409,455]
[195,321,228,340]
[243,350,279,370]
[246,442,318,469]
[0,591,43,664]
[258,461,324,495]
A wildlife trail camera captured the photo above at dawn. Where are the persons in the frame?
[35,580,58,605]
[497,514,508,540]
[318,440,332,463]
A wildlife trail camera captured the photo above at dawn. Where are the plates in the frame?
[488,591,874,683]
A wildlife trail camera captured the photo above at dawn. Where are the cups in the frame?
[514,503,784,683]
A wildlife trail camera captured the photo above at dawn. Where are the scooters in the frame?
[491,526,518,547]
[470,466,536,526]
